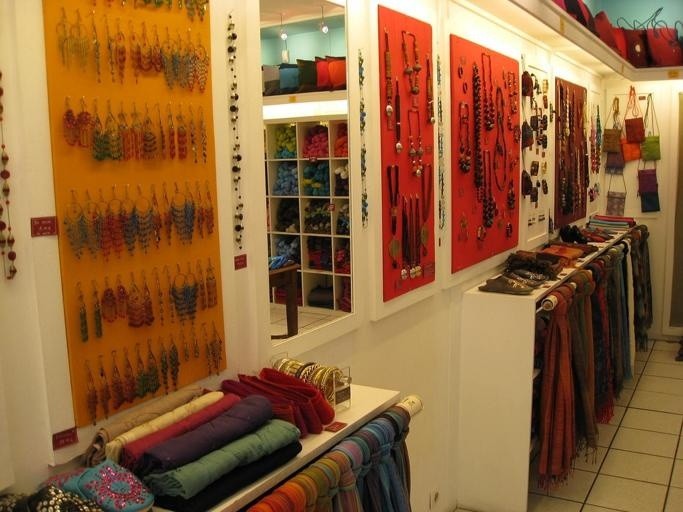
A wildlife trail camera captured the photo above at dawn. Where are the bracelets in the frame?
[273,356,346,406]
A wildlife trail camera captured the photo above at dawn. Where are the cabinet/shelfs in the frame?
[457,225,638,512]
[146,383,424,512]
[262,90,353,314]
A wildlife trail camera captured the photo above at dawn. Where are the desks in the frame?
[269,263,301,337]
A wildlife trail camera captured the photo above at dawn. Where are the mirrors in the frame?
[260,0,352,347]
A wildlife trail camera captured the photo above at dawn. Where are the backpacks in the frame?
[604,117,660,217]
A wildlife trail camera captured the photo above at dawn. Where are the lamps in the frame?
[279,13,288,41]
[320,5,328,34]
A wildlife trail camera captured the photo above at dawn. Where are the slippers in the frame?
[479,269,546,294]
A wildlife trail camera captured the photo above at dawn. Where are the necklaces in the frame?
[384,30,604,280]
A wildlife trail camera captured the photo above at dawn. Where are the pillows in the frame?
[262,57,345,95]
[553,0,622,56]
